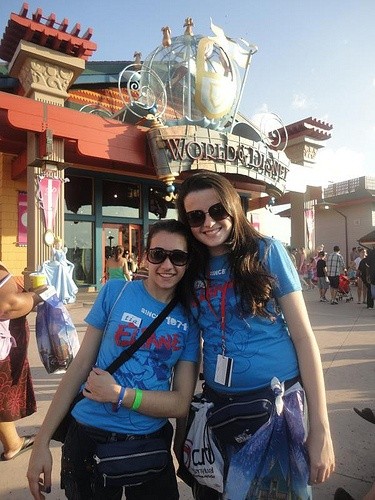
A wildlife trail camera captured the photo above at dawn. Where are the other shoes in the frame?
[363,302,367,304]
[312,285,316,289]
[306,286,311,291]
[368,306,372,310]
[358,300,360,304]
[320,297,327,302]
[330,300,338,304]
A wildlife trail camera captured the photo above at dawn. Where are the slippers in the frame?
[2,433,38,461]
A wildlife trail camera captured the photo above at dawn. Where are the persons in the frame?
[106,245,131,281]
[334,482,375,500]
[287,243,327,291]
[40,236,78,304]
[353,408,375,424]
[122,249,136,281]
[0,265,47,460]
[350,246,375,309]
[27,219,202,500]
[172,172,335,500]
[327,246,345,305]
[317,251,329,302]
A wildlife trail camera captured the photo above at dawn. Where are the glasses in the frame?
[145,247,191,267]
[180,203,230,226]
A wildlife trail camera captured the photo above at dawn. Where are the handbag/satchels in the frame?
[77,423,168,490]
[0,274,17,361]
[205,377,299,448]
[183,402,224,494]
[50,414,71,445]
[38,289,80,375]
[35,299,58,373]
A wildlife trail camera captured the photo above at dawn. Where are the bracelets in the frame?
[130,389,142,410]
[112,387,125,412]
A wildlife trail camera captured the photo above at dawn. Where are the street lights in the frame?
[108,235,114,257]
[324,205,349,277]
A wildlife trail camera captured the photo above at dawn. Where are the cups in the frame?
[30,273,46,305]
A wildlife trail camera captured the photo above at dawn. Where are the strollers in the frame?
[335,276,353,303]
